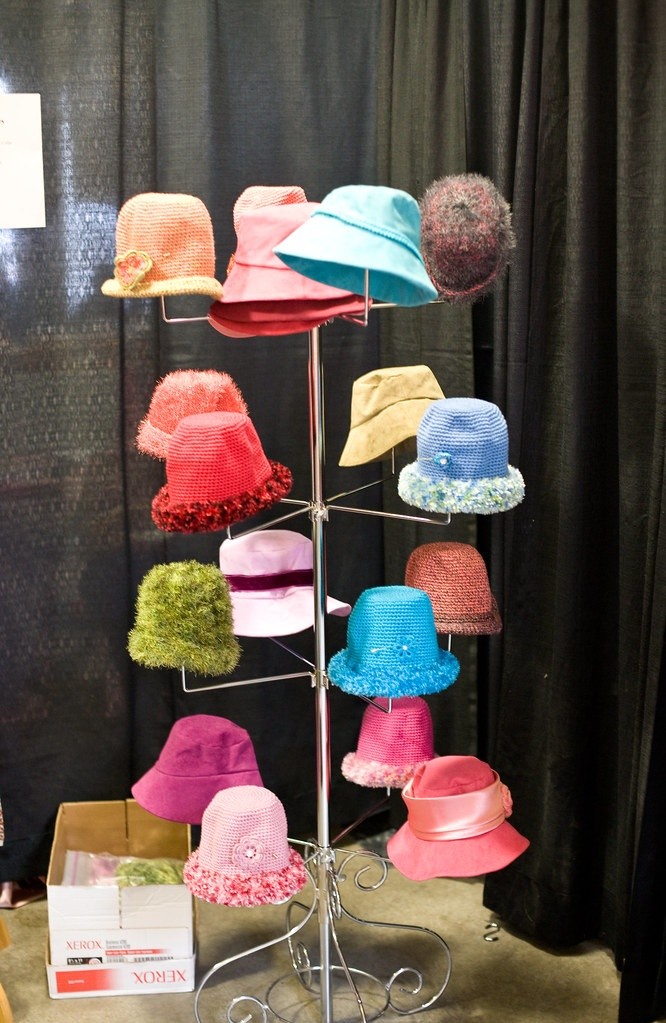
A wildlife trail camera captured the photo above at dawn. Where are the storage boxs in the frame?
[45,799,197,999]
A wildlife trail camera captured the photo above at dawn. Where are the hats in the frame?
[397,397,526,514]
[219,530,351,637]
[151,411,293,533]
[207,185,372,338]
[132,714,264,824]
[405,542,502,634]
[327,585,460,698]
[100,192,225,300]
[341,697,440,789]
[134,369,250,462]
[182,785,308,907]
[272,184,438,308]
[421,172,517,308]
[387,755,530,881]
[127,559,243,677]
[338,365,446,467]
